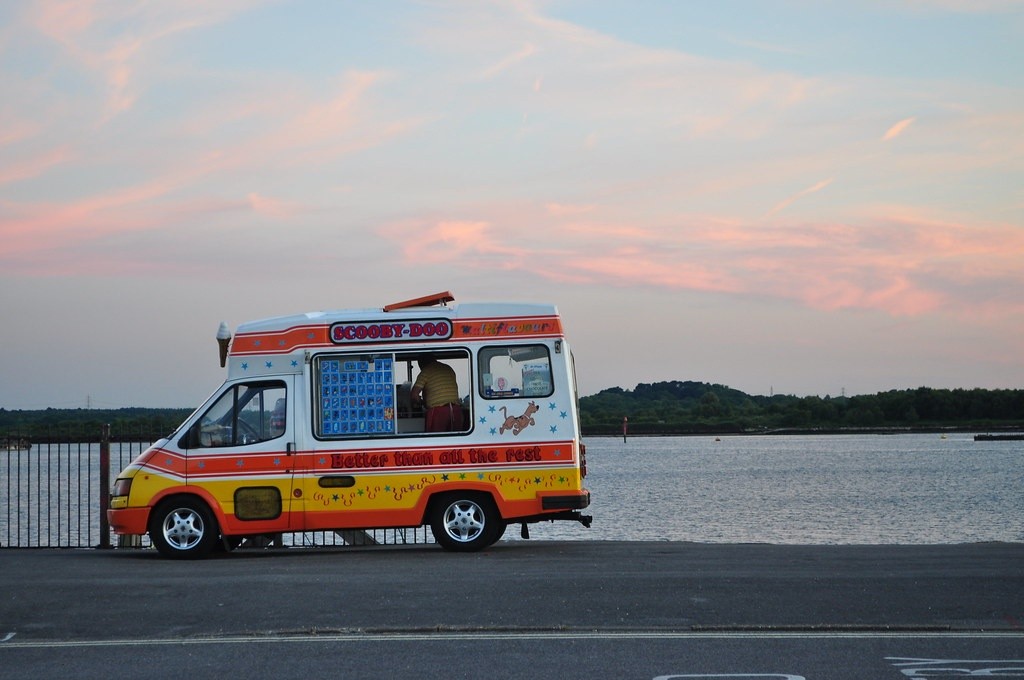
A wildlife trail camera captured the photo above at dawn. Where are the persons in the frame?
[411,359,462,432]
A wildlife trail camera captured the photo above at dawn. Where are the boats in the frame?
[973,432,1023,442]
[6,442,31,451]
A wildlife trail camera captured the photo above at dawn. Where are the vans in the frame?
[105,291,590,558]
